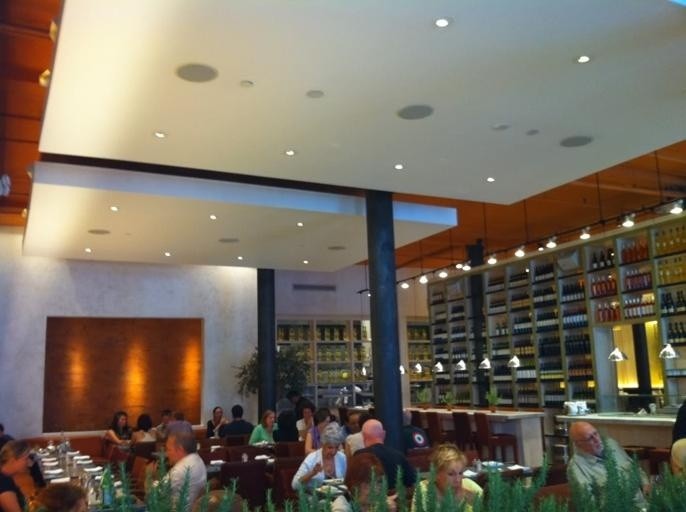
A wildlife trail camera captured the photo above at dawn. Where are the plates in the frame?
[461,457,533,477]
[33,432,123,490]
[254,453,272,461]
[209,458,228,467]
[316,476,349,495]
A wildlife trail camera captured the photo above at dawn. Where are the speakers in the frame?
[469,245,484,267]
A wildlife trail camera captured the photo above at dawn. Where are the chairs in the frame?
[410,410,518,464]
[338,408,349,426]
[210,436,568,510]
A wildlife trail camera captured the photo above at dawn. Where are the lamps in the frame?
[399,352,522,374]
[658,344,680,360]
[366,199,686,297]
[605,344,628,361]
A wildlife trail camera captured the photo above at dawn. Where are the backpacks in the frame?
[403,424,431,451]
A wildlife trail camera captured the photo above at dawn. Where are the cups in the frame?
[650,474,666,491]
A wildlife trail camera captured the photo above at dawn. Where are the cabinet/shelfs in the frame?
[275,315,376,407]
[406,316,433,400]
[425,210,622,411]
[649,217,686,408]
[582,224,656,323]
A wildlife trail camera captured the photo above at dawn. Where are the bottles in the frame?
[549,414,570,464]
[654,222,686,346]
[590,236,655,322]
[432,262,597,415]
[277,319,372,386]
[406,325,433,406]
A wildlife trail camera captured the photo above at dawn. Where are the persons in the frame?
[567,421,650,510]
[671,394,686,476]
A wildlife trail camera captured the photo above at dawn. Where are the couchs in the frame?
[28,425,212,475]
